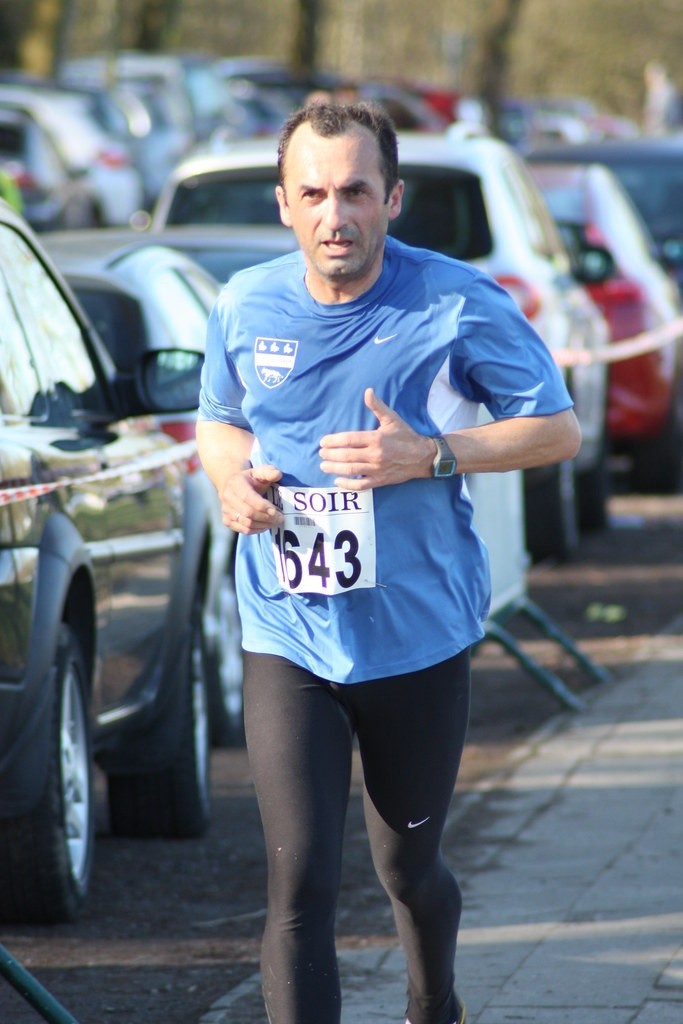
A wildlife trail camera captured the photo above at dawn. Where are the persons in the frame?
[196,100,581,1024]
[296,54,683,143]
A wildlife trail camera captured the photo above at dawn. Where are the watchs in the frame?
[427,435,459,479]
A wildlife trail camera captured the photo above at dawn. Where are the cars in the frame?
[1,42,683,930]
